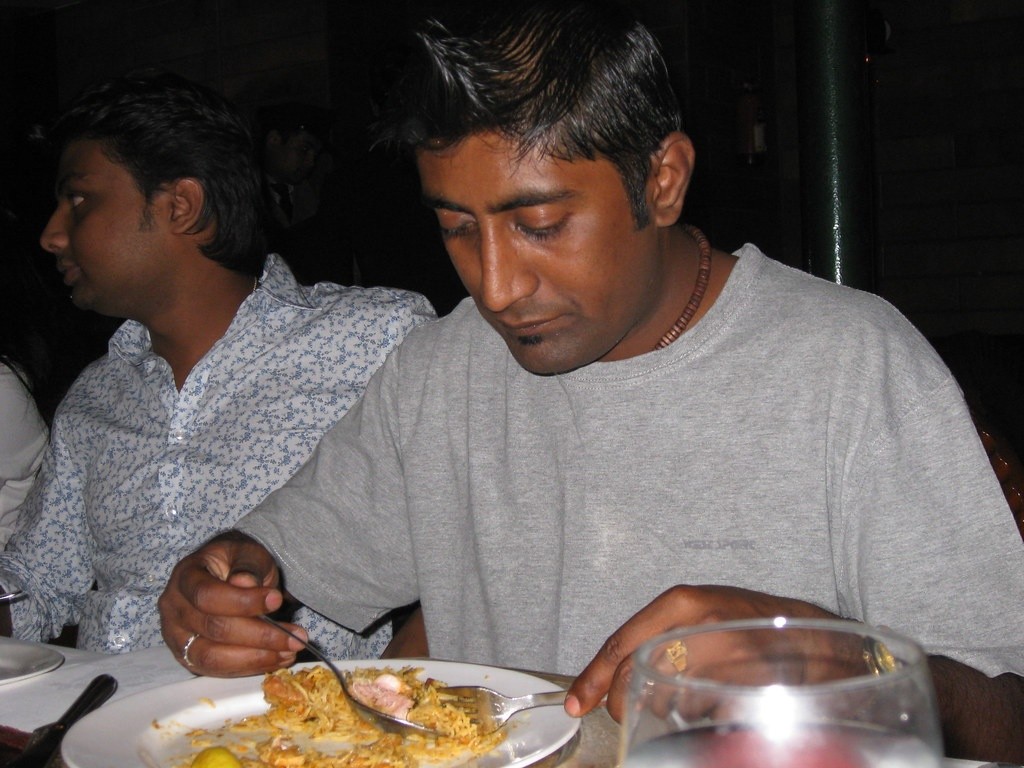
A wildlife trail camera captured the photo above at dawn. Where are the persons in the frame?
[159,1,1024,768]
[0,71,437,662]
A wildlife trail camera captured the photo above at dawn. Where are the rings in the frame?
[182,633,200,666]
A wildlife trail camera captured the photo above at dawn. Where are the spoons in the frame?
[203,564,433,732]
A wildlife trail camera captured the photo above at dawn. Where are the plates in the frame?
[61,660,582,768]
[0,636,64,685]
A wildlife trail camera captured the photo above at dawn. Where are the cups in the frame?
[617,619,943,768]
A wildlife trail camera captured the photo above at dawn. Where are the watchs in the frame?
[863,625,927,732]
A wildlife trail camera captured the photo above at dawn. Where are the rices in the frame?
[181,666,505,768]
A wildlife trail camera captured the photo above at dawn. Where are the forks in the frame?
[435,686,608,735]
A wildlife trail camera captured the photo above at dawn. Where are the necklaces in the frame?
[652,222,712,351]
